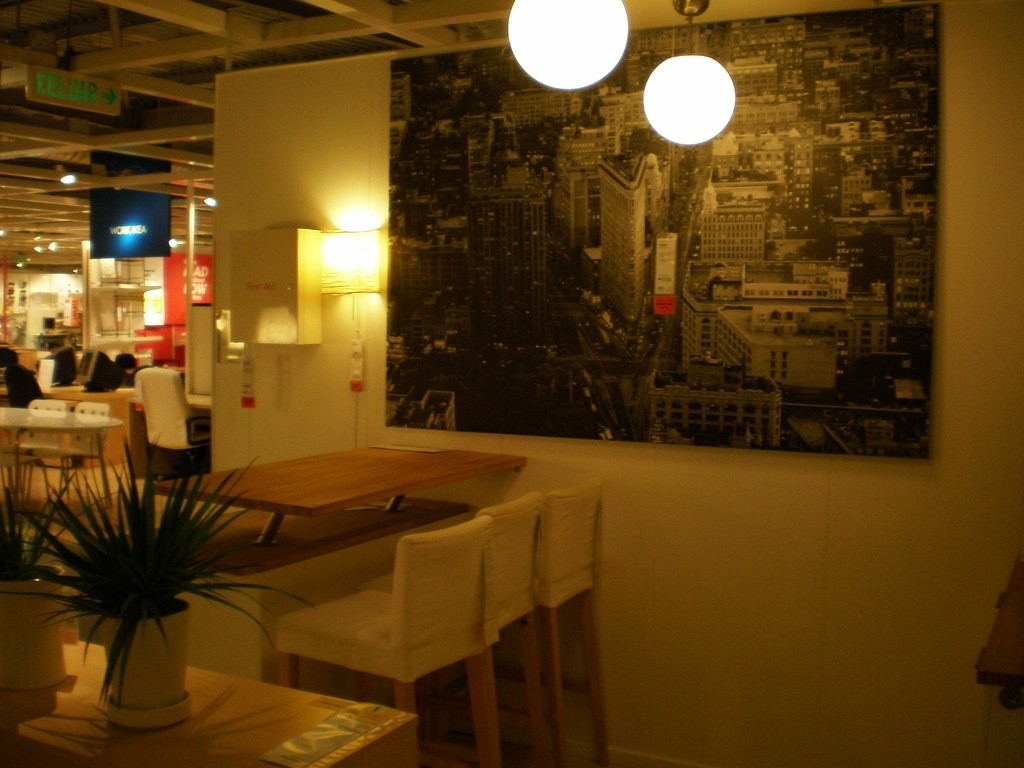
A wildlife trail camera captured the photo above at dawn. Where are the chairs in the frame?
[271,513,506,768]
[0,400,109,504]
[357,476,608,768]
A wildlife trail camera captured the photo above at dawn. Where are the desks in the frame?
[144,443,529,578]
[122,394,212,483]
[0,631,427,767]
[0,408,125,505]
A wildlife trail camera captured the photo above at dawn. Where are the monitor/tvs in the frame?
[42,317,56,332]
[75,348,125,392]
[36,345,75,387]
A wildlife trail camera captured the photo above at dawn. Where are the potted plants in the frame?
[0,438,88,718]
[0,424,322,730]
